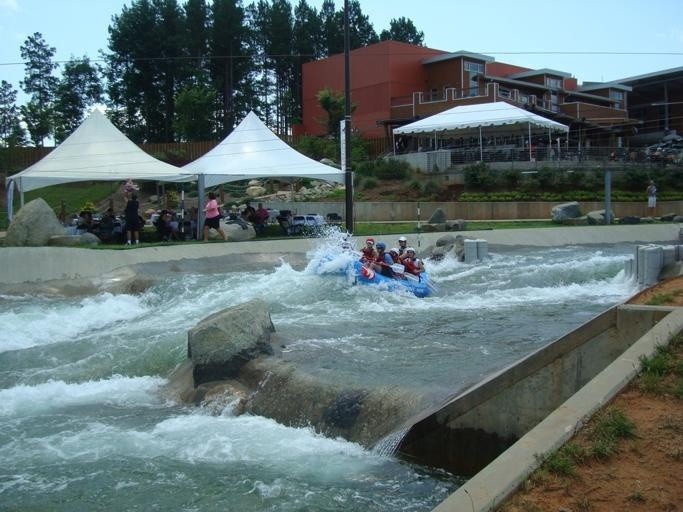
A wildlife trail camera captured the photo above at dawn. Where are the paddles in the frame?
[421,274,441,293]
[362,259,404,274]
[403,271,419,281]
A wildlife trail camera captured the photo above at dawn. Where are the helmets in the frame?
[407,248,415,254]
[376,242,385,250]
[399,237,407,246]
[366,239,374,245]
[390,248,399,256]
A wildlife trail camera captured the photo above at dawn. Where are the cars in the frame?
[292,214,327,235]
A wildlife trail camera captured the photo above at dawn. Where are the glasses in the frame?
[407,251,414,254]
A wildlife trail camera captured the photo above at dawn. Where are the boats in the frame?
[345,261,428,298]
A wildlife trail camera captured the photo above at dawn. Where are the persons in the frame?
[75,206,199,243]
[394,135,683,166]
[241,200,257,227]
[357,235,426,282]
[255,202,268,226]
[646,180,657,219]
[122,191,140,247]
[200,191,228,245]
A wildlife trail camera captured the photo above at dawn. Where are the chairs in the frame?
[152,221,173,241]
[264,208,343,236]
